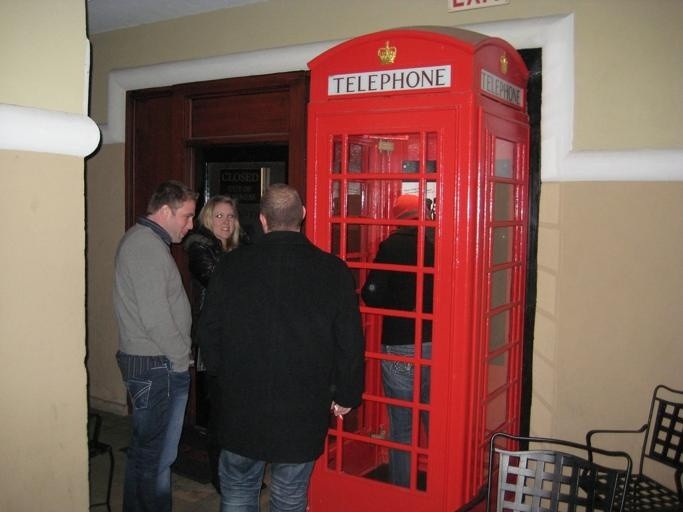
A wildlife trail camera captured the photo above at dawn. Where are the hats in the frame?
[394,193,428,220]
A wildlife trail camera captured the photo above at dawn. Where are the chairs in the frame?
[487,384,683,512]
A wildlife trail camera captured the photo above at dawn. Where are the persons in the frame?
[194,183,365,511]
[110,178,199,512]
[181,194,266,496]
[359,194,435,488]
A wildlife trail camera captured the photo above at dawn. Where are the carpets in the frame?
[119,423,214,485]
